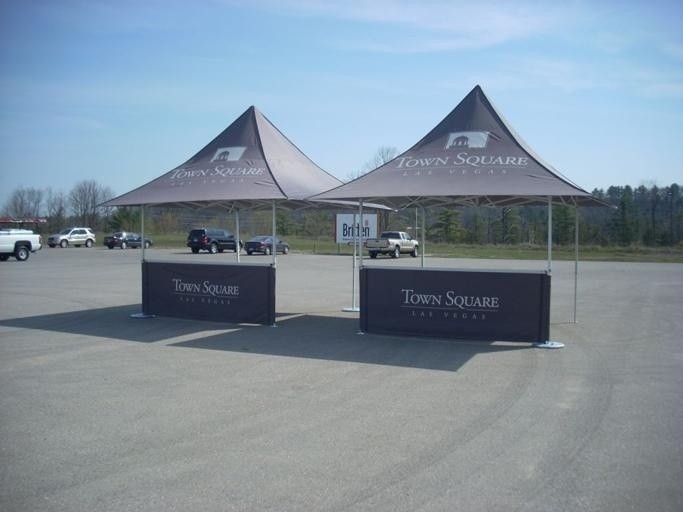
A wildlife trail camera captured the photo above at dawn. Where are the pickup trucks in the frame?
[365,231,419,259]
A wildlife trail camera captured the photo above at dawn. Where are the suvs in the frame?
[47,227,97,248]
[186,228,243,254]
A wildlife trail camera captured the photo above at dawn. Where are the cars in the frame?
[244,235,290,255]
[0,228,43,261]
[103,231,153,249]
